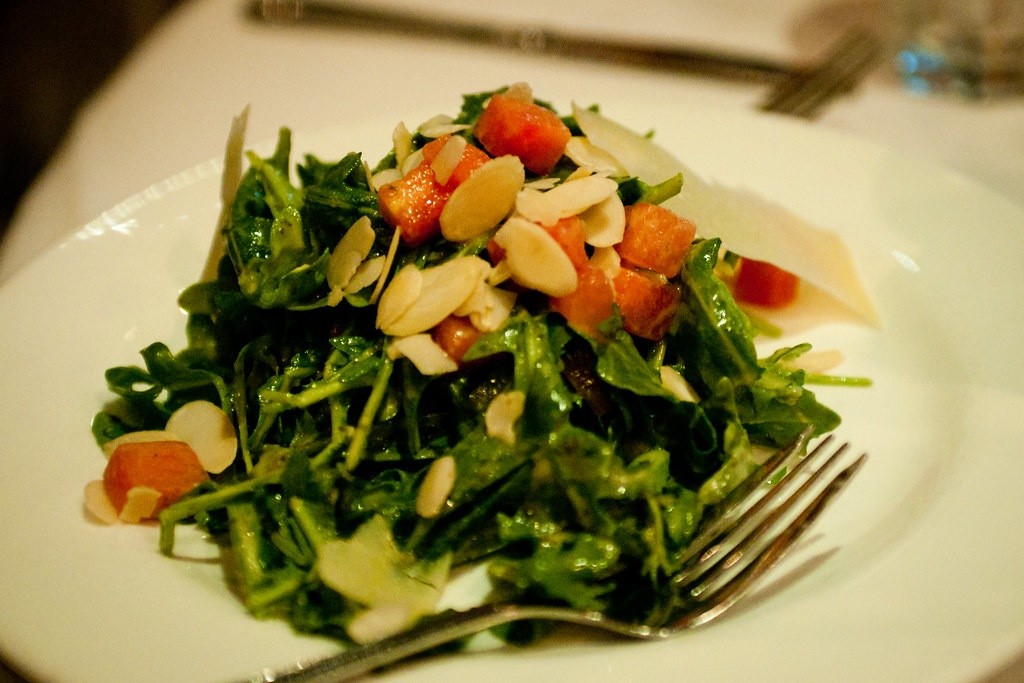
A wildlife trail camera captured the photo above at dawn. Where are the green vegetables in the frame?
[90,89,878,666]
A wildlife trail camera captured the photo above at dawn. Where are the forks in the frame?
[753,24,888,118]
[245,423,869,683]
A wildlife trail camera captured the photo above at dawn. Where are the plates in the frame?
[304,0,818,68]
[2,104,1023,683]
